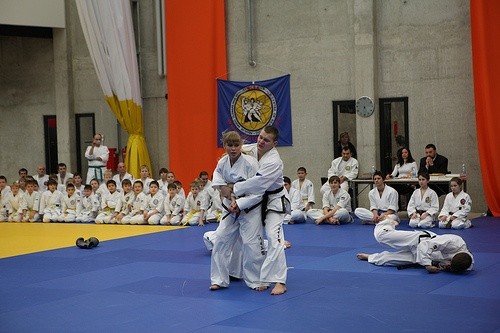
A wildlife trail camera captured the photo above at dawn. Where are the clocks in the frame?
[355,96,375,117]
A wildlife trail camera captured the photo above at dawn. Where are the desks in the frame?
[350,176,466,212]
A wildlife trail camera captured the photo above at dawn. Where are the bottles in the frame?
[411,167,416,178]
[461,164,466,174]
[370,166,376,180]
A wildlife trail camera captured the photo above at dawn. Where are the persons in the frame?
[228,125,287,295]
[320,147,359,196]
[386,147,419,211]
[209,131,270,291]
[356,219,475,273]
[338,132,357,159]
[84,133,110,185]
[0,163,473,227]
[418,144,449,197]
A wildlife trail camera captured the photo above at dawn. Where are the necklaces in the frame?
[378,189,384,192]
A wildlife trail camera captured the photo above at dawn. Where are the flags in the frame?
[217,74,293,148]
